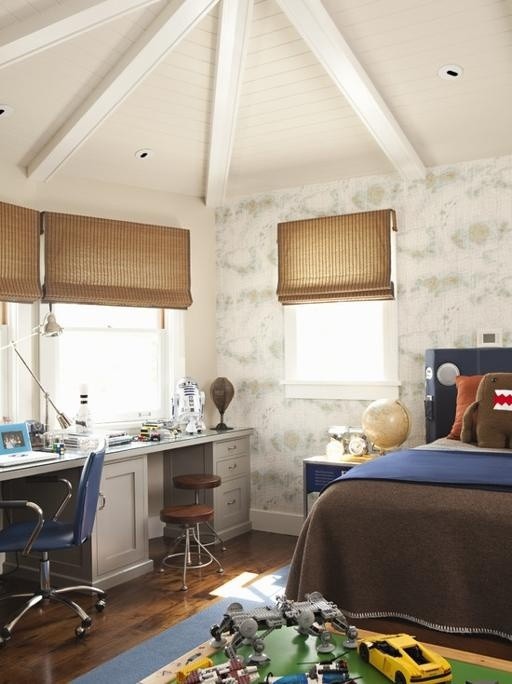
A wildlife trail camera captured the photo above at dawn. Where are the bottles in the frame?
[75,392,94,433]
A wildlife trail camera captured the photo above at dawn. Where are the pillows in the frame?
[446,375,484,440]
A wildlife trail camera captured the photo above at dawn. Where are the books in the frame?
[0,449,61,468]
[62,427,134,454]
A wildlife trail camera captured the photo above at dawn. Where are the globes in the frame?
[360,399,412,457]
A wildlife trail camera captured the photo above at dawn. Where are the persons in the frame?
[2,431,25,449]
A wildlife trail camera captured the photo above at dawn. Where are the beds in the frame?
[285,437,512,661]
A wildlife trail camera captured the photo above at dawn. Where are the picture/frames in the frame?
[0,422,33,455]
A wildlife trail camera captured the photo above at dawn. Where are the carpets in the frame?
[67,557,512,684]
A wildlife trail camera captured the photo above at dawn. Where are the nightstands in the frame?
[302,455,370,525]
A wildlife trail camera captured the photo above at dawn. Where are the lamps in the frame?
[349,434,368,457]
[10,302,72,430]
[210,377,234,430]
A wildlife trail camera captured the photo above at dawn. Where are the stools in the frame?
[158,503,223,591]
[173,473,221,565]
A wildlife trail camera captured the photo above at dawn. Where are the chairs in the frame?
[0,438,108,640]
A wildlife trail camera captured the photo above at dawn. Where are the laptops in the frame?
[0,421,60,466]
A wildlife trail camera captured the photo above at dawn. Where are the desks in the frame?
[0,427,254,598]
